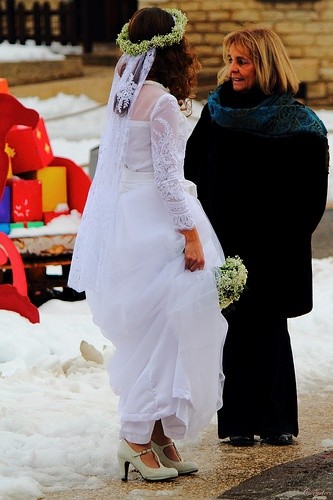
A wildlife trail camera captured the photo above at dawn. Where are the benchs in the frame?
[0,235,90,301]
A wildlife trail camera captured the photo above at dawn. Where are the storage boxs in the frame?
[0,118,71,236]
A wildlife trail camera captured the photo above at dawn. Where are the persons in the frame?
[184,25,329,447]
[67,6,230,482]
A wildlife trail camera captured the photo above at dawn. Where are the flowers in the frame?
[115,8,188,57]
[217,254,248,309]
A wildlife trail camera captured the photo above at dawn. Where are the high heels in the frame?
[150,438,198,475]
[117,438,178,482]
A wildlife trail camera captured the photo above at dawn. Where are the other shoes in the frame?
[230,435,254,446]
[262,434,292,445]
